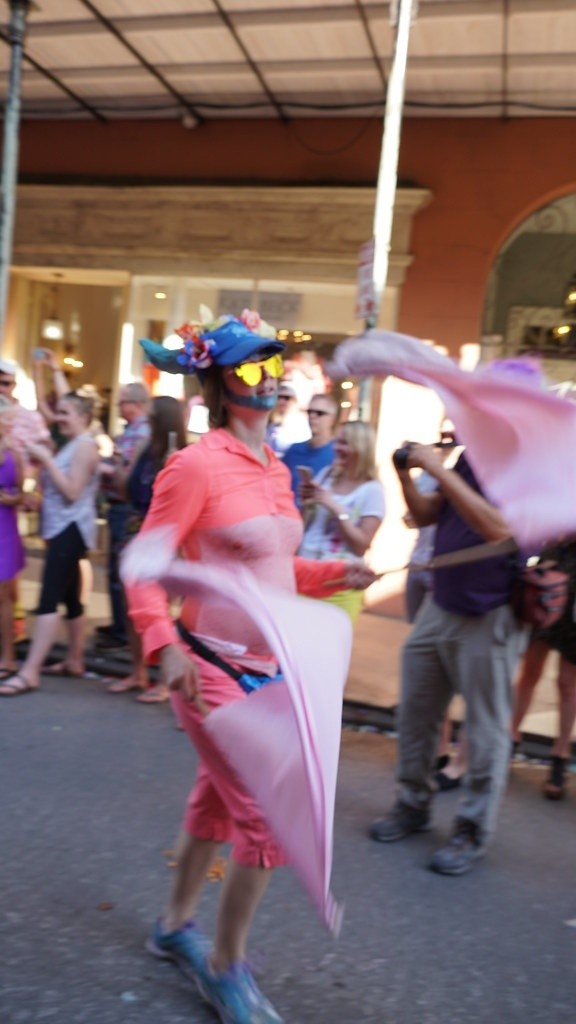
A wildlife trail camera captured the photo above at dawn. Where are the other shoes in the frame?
[366,802,432,843]
[430,818,486,874]
[97,625,123,638]
[94,634,131,654]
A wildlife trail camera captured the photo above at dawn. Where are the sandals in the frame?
[542,756,569,801]
[433,768,467,794]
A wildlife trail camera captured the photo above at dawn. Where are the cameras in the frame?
[393,442,416,468]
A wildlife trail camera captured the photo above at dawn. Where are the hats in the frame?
[136,319,284,370]
[0,359,17,376]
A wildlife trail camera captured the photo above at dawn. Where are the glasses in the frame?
[220,353,282,385]
[275,394,294,404]
[0,380,14,387]
[304,409,332,419]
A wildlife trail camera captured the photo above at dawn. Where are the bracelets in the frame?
[336,512,348,523]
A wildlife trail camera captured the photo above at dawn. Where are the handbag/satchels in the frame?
[242,666,285,693]
[513,566,568,627]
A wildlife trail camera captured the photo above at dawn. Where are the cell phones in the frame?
[296,465,312,485]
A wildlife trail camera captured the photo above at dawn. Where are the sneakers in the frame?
[138,913,219,1003]
[196,947,283,1024]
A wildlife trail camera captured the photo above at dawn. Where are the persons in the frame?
[368,360,576,877]
[120,336,377,1024]
[288,384,384,624]
[0,358,208,729]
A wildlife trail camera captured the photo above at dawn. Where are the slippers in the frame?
[105,678,145,692]
[0,666,19,678]
[137,691,171,705]
[43,664,83,677]
[0,673,38,696]
